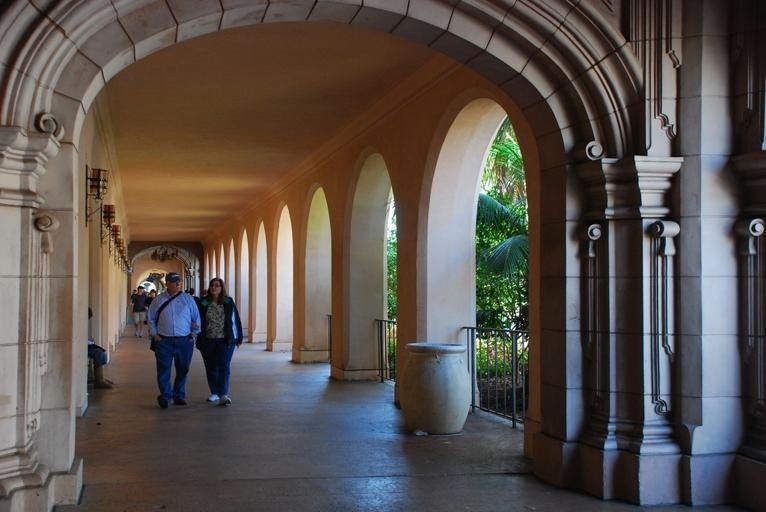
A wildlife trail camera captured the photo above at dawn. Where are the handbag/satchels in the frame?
[150,334,158,352]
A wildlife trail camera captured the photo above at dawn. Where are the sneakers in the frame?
[173,398,187,406]
[134,331,144,339]
[93,377,115,390]
[157,395,169,409]
[207,393,220,402]
[219,395,232,406]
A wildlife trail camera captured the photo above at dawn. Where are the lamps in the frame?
[84,163,133,274]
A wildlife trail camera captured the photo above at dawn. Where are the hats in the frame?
[137,286,145,290]
[165,273,180,283]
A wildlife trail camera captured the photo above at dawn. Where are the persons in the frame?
[191,277,243,405]
[146,271,202,409]
[87,306,114,390]
[127,282,208,338]
